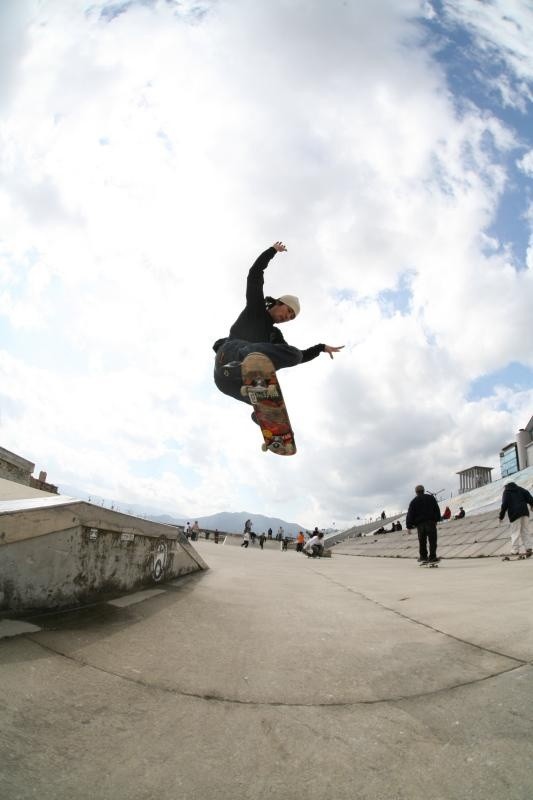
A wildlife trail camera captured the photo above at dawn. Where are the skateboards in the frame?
[240,350,297,457]
[305,552,323,560]
[417,559,440,568]
[498,551,531,562]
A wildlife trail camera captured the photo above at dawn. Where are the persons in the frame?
[441,506,451,520]
[405,485,442,564]
[498,478,533,554]
[210,241,346,426]
[380,511,386,518]
[182,518,404,559]
[454,507,465,519]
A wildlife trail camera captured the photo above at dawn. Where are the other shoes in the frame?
[222,361,242,379]
[251,412,259,425]
[526,549,532,552]
[430,557,439,563]
[421,557,429,561]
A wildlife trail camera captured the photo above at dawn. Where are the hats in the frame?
[280,295,300,319]
[503,479,514,486]
